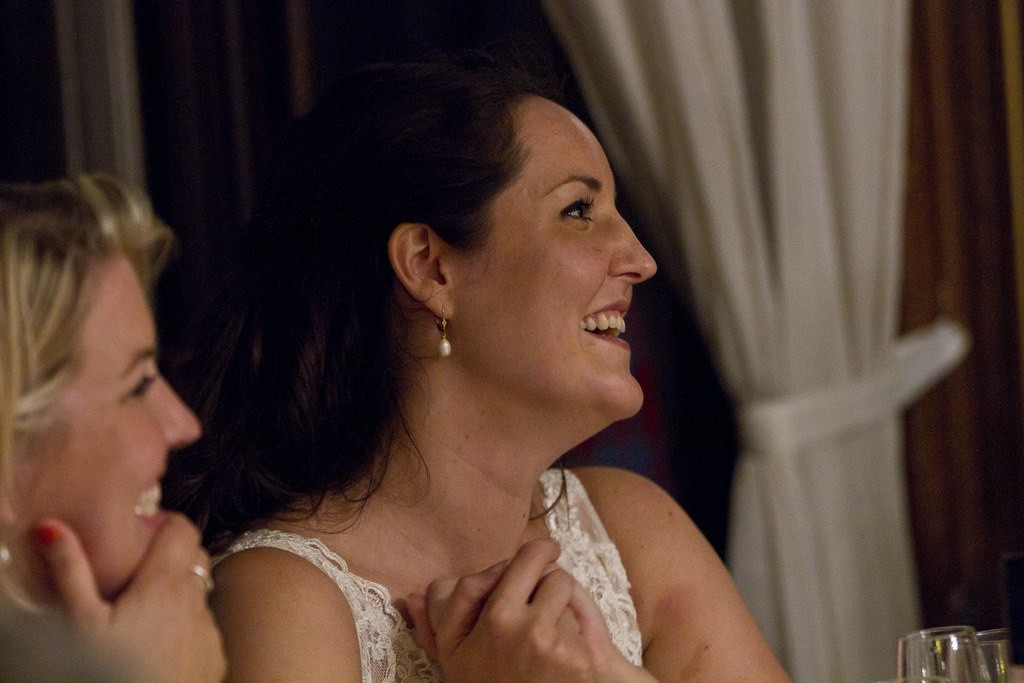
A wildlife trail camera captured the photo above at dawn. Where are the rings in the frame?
[193,566,215,592]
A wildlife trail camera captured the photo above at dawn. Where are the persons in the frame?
[183,56,793,683]
[0,170,229,683]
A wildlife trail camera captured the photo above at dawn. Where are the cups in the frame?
[974,628,1013,683]
[895,625,992,683]
